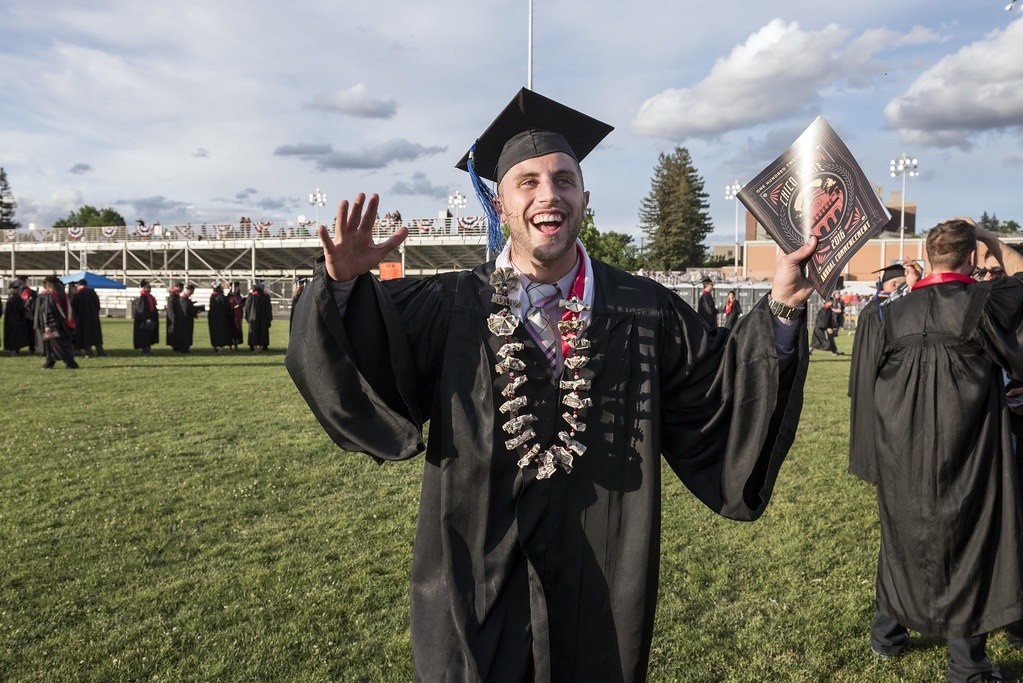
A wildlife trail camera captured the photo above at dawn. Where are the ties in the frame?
[522,282,560,379]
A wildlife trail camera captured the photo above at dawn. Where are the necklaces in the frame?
[486,265,593,480]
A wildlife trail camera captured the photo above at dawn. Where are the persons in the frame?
[284,85,818,682]
[697,218,1022,683]
[0,212,401,369]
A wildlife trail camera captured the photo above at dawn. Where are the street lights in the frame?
[724,181,747,280]
[309,186,328,231]
[890,154,918,261]
[448,190,466,217]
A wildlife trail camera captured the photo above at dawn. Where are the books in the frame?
[734,120,891,301]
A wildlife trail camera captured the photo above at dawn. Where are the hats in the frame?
[872,262,907,300]
[174,281,183,287]
[292,278,308,285]
[140,279,149,287]
[186,285,195,289]
[10,275,88,291]
[211,279,266,292]
[456,87,616,250]
[700,275,710,282]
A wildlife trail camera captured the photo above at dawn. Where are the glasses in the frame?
[978,268,1004,280]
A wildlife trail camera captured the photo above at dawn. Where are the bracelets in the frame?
[769,293,805,321]
